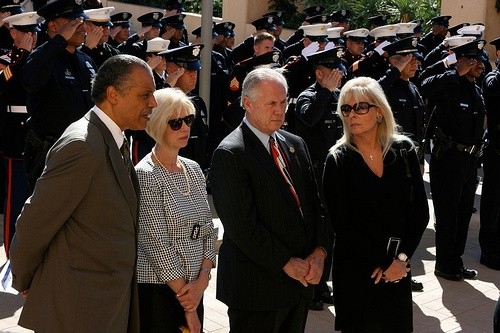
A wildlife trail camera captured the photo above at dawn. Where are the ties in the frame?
[269,137,302,209]
[122,137,131,175]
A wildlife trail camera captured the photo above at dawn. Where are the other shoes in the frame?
[411,278,424,291]
[309,281,335,311]
[434,266,477,281]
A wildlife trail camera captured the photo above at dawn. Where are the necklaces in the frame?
[361,142,380,161]
[152,145,191,196]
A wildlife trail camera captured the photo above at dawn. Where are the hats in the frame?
[158,43,206,71]
[37,0,89,22]
[160,13,187,30]
[136,11,164,28]
[2,11,46,32]
[109,12,133,27]
[83,6,115,27]
[1,5,23,16]
[146,37,170,54]
[194,5,500,72]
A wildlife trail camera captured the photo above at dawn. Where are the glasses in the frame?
[168,113,195,131]
[340,101,378,117]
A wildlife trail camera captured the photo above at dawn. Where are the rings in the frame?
[395,280,399,283]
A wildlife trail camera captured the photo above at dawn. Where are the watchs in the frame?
[395,253,410,263]
[200,268,211,280]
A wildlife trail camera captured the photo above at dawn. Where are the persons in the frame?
[0,0,500,333]
